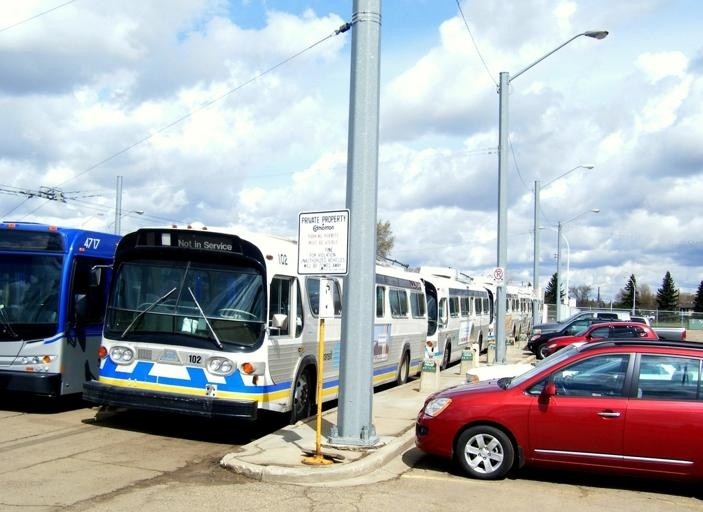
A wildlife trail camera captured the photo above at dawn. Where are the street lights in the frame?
[532,164,595,326]
[495,29,609,363]
[624,276,635,316]
[80,209,144,230]
[538,208,600,323]
